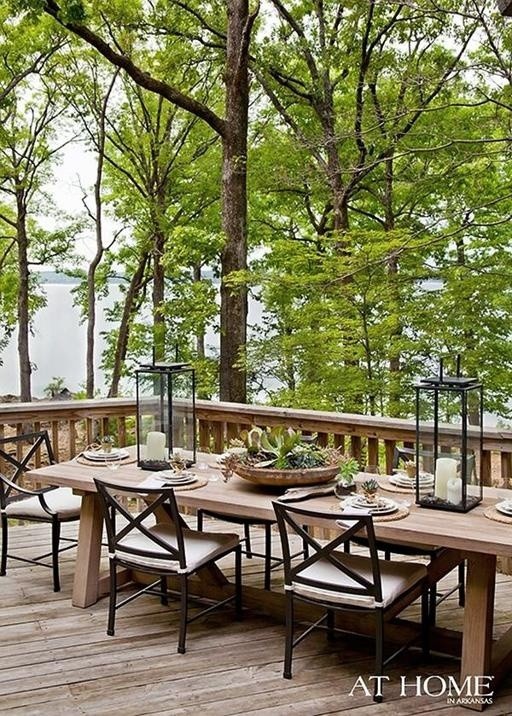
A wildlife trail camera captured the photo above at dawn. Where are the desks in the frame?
[25,443,512,711]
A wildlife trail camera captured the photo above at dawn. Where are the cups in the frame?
[104,447,120,471]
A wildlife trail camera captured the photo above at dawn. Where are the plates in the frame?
[494,498,512,518]
[387,469,435,489]
[83,448,130,461]
[150,469,199,485]
[338,496,400,515]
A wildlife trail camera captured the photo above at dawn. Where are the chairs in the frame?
[0,428,512,703]
